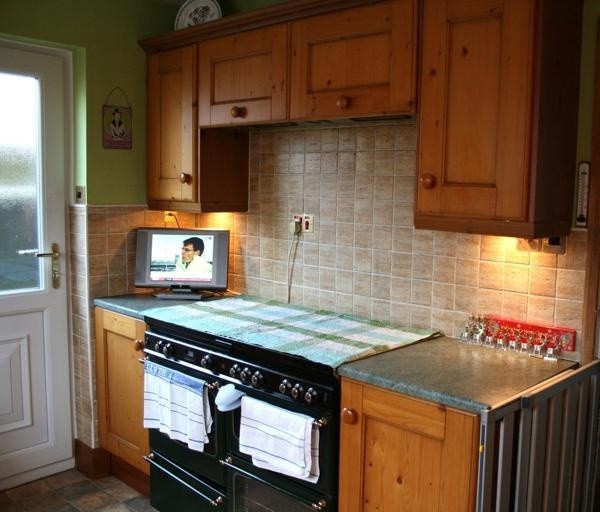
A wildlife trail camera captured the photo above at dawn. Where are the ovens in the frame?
[143,327,339,512]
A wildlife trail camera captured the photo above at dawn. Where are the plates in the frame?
[173,0,223,31]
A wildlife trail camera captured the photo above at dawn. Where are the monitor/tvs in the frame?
[135,226,229,301]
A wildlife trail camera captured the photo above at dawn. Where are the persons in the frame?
[109,108,126,138]
[175,237,212,278]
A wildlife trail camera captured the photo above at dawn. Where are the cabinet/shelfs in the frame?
[413,0,584,239]
[338,375,579,511]
[95,306,150,478]
[198,1,419,127]
[147,46,249,214]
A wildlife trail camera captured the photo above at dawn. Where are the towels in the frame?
[239,396,321,485]
[143,360,214,453]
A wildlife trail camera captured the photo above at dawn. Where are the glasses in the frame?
[182,247,193,251]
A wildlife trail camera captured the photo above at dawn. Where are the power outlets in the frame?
[288,213,314,234]
[164,210,178,222]
